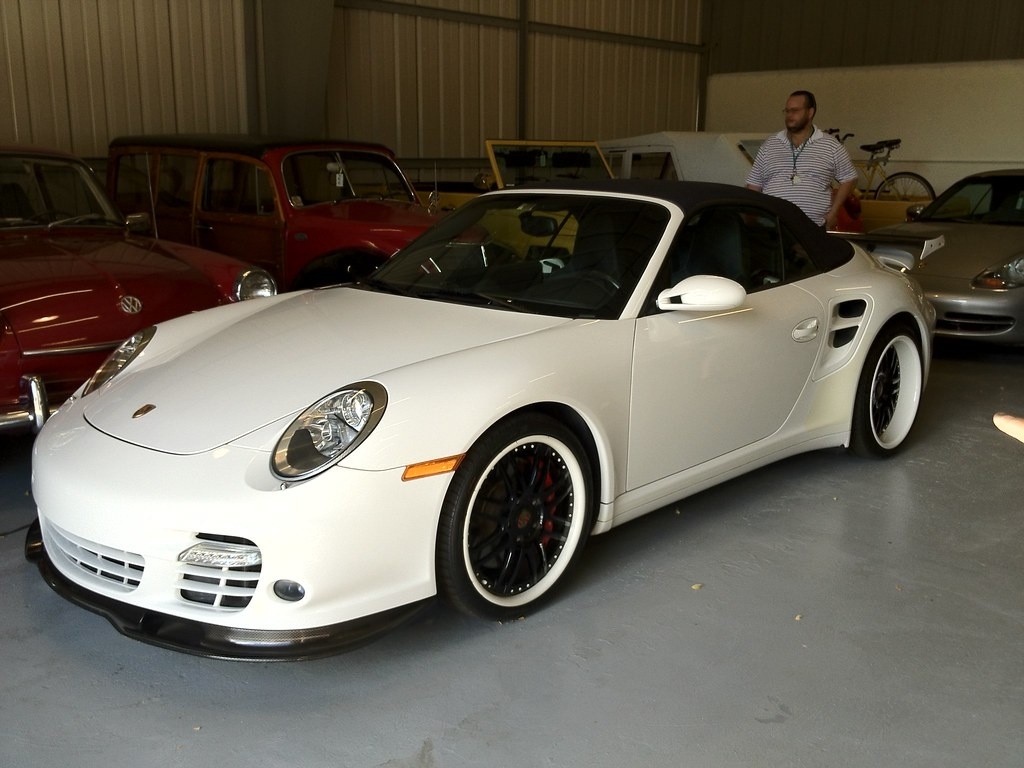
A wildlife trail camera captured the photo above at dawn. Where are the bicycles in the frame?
[823,125,938,203]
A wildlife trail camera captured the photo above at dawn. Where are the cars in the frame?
[104,132,493,292]
[372,139,619,265]
[563,129,781,189]
[1,143,279,433]
[855,168,1024,346]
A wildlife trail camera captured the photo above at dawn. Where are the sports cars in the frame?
[22,176,938,665]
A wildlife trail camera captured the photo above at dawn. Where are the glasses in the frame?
[781,106,810,115]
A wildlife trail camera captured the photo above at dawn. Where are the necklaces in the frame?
[787,127,815,185]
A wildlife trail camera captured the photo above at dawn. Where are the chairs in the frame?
[574,200,675,320]
[0,183,42,223]
[665,207,753,299]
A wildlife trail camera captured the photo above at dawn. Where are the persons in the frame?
[747,91,859,233]
